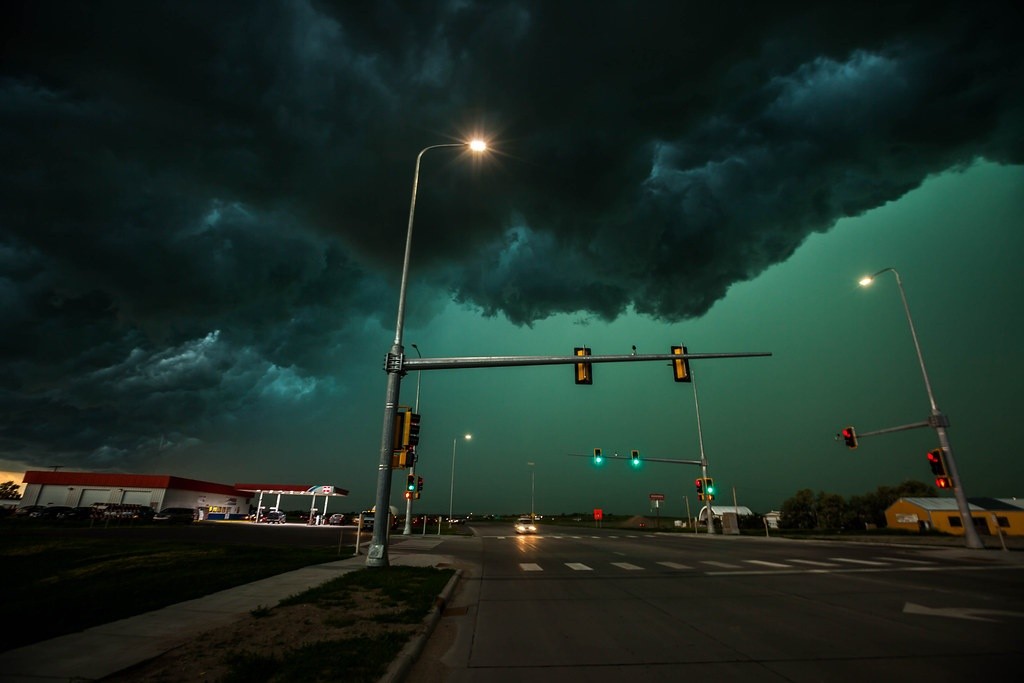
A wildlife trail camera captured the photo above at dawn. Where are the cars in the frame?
[329,513,345,525]
[514,517,536,534]
[13,504,198,528]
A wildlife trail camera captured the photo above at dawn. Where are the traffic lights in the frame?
[405,492,411,498]
[406,474,415,492]
[842,427,859,449]
[708,494,715,500]
[705,478,714,494]
[927,448,947,476]
[415,475,423,492]
[695,479,704,494]
[935,477,953,489]
[416,493,420,499]
[402,411,421,446]
[631,450,640,465]
[698,494,704,501]
[399,451,415,467]
[594,448,603,464]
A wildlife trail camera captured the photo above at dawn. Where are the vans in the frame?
[266,510,287,524]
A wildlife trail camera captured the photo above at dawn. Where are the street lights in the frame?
[403,342,422,535]
[858,267,985,549]
[447,434,472,529]
[363,139,488,566]
